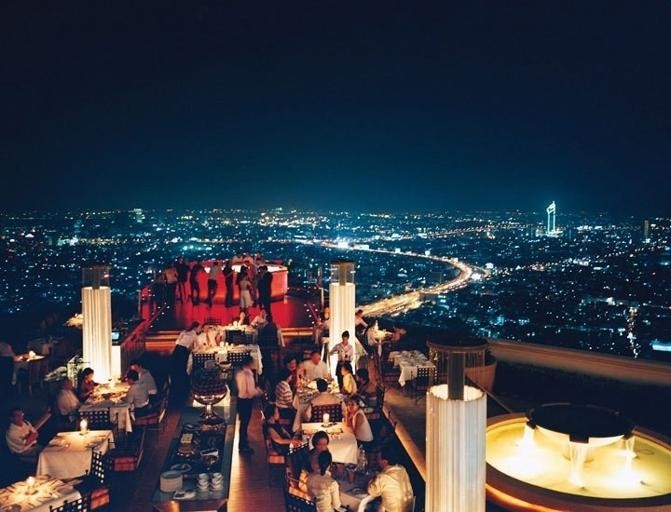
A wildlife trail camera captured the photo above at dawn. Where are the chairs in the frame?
[0,318,440,512]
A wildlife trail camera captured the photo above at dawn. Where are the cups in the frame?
[198,473,224,491]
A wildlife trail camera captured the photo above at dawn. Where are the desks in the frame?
[79,382,130,424]
[301,421,358,465]
[152,378,237,511]
[388,350,436,387]
[36,430,115,480]
[1,474,82,512]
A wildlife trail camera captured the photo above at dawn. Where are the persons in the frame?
[233,356,262,456]
[77,368,96,402]
[172,321,211,375]
[6,405,45,457]
[236,308,278,362]
[354,309,416,352]
[122,358,161,417]
[57,376,81,425]
[164,251,273,315]
[366,445,414,512]
[269,331,376,455]
[293,431,341,512]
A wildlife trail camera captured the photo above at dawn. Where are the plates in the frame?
[160,471,184,494]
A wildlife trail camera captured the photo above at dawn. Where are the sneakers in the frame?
[240,445,254,454]
